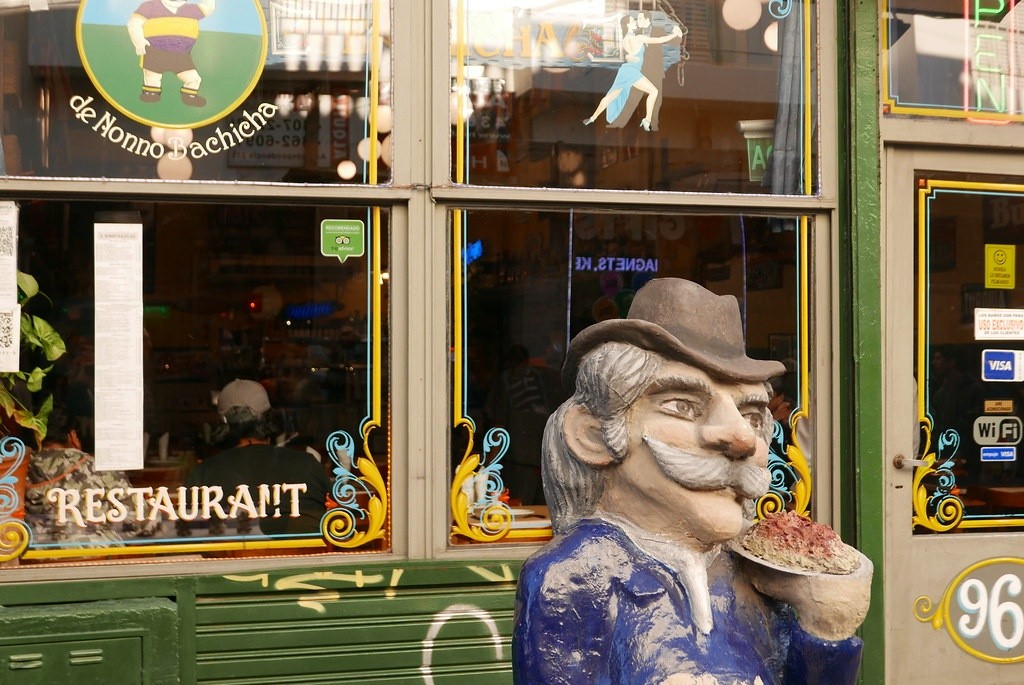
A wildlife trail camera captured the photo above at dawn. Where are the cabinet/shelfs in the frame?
[143,317,390,464]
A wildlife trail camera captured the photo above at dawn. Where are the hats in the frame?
[216,378,271,418]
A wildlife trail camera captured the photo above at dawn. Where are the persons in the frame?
[487,346,562,468]
[930,342,1009,467]
[26,412,163,549]
[767,370,801,503]
[511,276,874,685]
[331,325,366,406]
[181,379,366,540]
